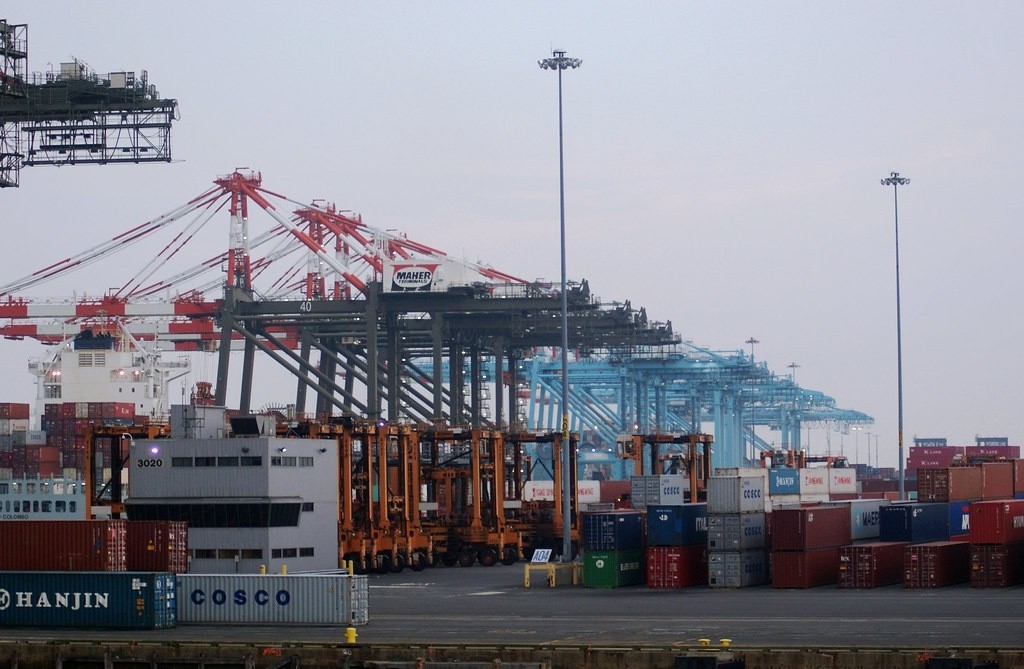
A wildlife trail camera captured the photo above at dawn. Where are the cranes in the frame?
[0,171,684,426]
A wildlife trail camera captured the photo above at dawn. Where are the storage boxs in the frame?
[523,446,1023,588]
[2,401,368,635]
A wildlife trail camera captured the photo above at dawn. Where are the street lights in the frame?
[746,337,760,470]
[535,49,583,561]
[881,172,911,499]
[787,363,800,450]
